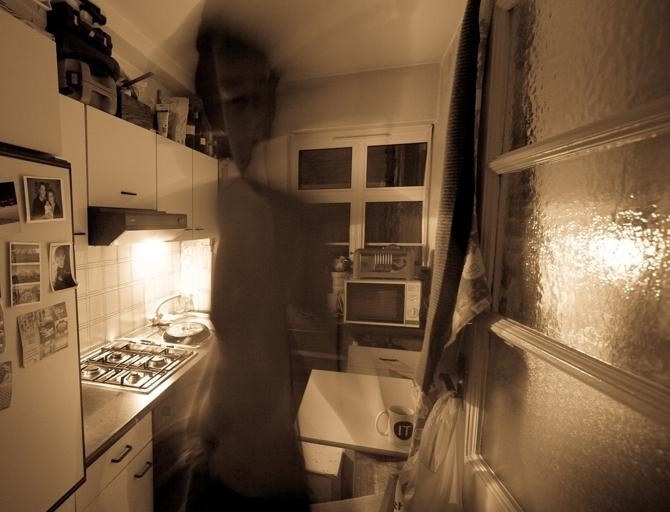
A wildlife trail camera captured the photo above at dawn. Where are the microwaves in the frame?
[342,276,423,331]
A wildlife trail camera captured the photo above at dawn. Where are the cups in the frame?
[375,404,416,448]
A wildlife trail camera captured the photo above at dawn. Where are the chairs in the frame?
[348,342,421,379]
[310,469,399,512]
[301,442,345,502]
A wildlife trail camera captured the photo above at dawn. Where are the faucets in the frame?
[156,293,182,317]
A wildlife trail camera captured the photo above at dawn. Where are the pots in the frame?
[149,321,212,346]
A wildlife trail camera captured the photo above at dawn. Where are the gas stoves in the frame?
[77,339,202,396]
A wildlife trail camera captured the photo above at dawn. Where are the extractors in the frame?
[87,204,189,251]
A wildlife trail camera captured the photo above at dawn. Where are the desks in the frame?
[280,323,426,416]
[295,368,420,498]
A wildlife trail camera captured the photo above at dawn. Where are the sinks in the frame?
[172,312,210,331]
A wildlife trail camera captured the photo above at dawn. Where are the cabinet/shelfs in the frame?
[56,408,153,512]
[59,93,218,242]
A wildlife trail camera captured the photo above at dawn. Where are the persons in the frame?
[53,246,76,290]
[32,182,60,220]
